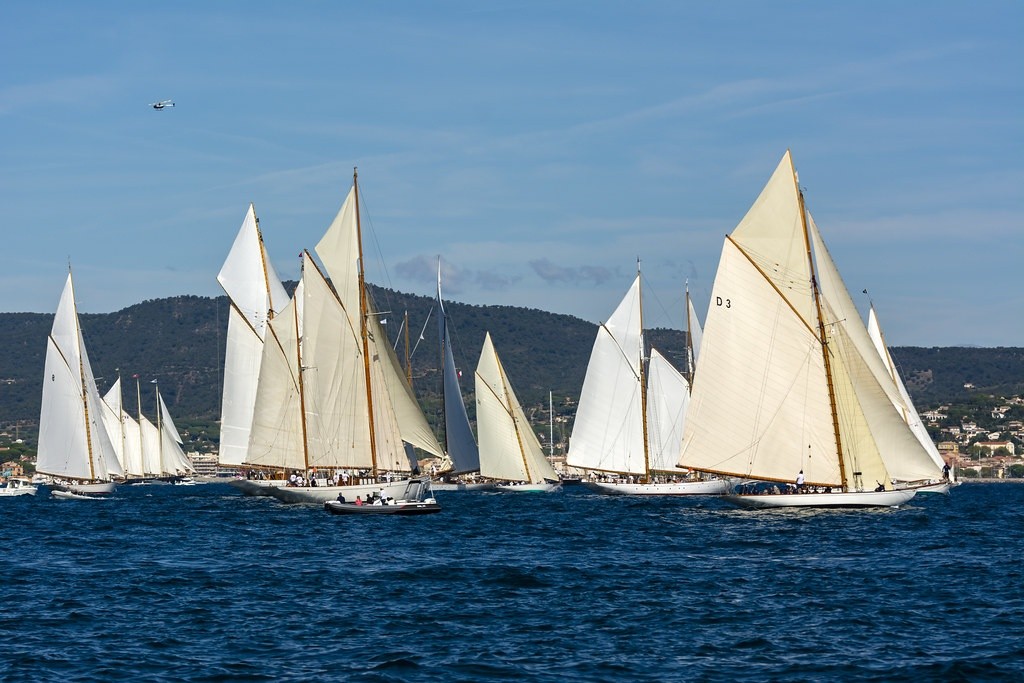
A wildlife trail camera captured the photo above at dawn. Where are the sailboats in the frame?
[474,329,561,494]
[564,255,734,499]
[0,140,957,515]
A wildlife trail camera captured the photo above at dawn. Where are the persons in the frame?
[356,496,362,507]
[336,493,345,504]
[589,472,677,484]
[246,469,264,480]
[288,469,350,487]
[796,470,804,494]
[942,462,951,480]
[367,487,387,505]
